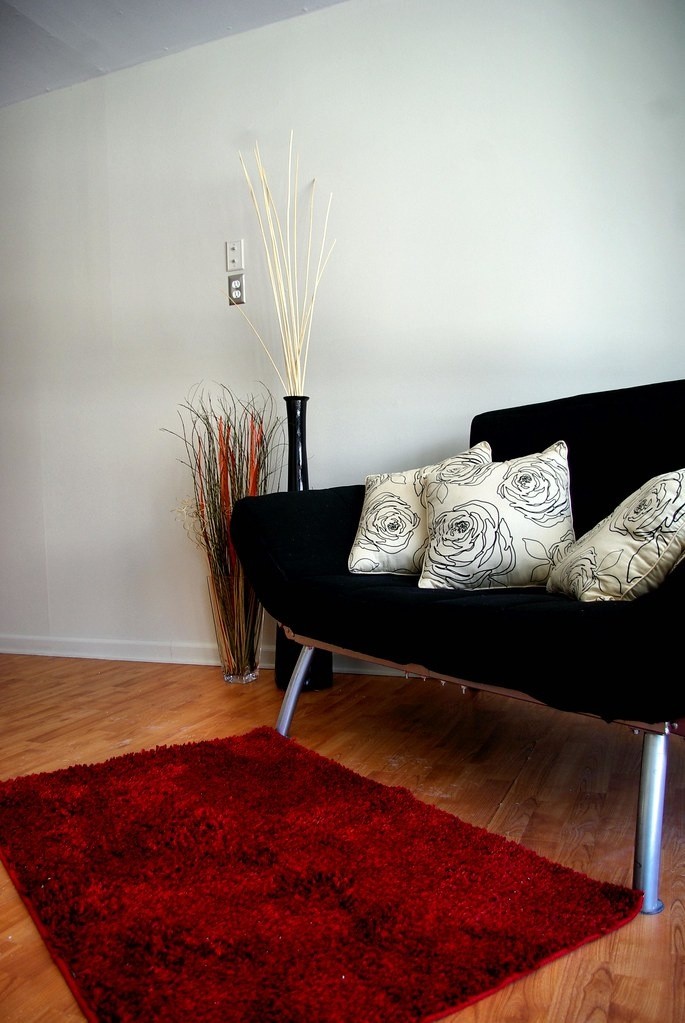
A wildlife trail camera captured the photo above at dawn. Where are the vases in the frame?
[274,396,334,692]
[204,575,264,684]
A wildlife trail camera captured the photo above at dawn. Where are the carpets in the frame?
[0,726,644,1023]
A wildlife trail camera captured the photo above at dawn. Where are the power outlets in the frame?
[228,273,245,306]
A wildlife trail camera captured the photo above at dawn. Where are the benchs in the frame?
[228,379,685,915]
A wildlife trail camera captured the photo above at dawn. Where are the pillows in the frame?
[418,440,576,590]
[545,469,685,603]
[347,441,492,577]
